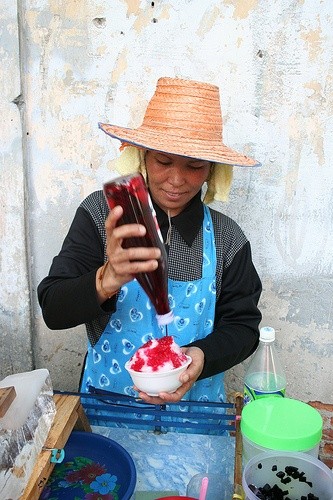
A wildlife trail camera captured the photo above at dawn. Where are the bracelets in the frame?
[99,261,119,299]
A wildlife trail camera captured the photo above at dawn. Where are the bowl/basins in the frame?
[38,431,137,500]
[125,355,193,397]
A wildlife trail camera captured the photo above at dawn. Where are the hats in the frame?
[98,77,256,167]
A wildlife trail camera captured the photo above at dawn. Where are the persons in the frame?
[37,78,263,500]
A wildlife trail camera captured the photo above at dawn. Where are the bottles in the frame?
[242,326,286,407]
[102,172,175,326]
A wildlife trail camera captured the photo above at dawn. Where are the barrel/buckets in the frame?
[242,450,333,500]
[240,397,323,466]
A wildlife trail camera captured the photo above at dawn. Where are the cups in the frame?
[187,472,234,500]
[156,495,199,500]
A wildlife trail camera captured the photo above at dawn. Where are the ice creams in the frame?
[126,334,187,374]
